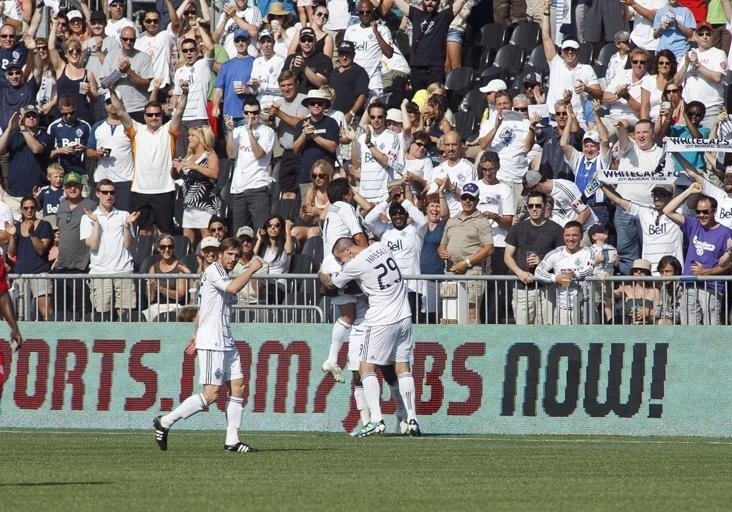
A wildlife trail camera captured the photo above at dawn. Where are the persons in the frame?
[318,237,421,438]
[152,238,262,453]
[320,179,367,384]
[0,0,732,324]
[0,256,23,350]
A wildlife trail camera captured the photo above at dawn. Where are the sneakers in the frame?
[320,360,345,384]
[357,419,386,439]
[223,442,259,452]
[349,421,374,438]
[153,414,170,450]
[399,418,422,437]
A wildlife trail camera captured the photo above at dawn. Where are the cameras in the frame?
[104,149,111,157]
[302,204,308,216]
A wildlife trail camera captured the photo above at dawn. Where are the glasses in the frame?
[160,244,174,250]
[527,203,542,208]
[482,168,497,173]
[0,34,16,39]
[695,208,713,214]
[514,107,527,112]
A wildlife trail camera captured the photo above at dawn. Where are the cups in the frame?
[104,148,112,157]
[526,250,534,268]
[571,73,582,95]
[561,271,569,288]
[662,102,670,113]
[271,20,280,32]
[630,304,638,318]
[79,82,87,94]
[234,81,242,93]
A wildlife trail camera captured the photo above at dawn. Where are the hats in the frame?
[521,169,543,196]
[199,236,221,249]
[651,184,673,195]
[478,78,507,94]
[521,20,714,145]
[6,0,480,230]
[235,225,255,239]
[631,259,651,271]
[588,224,606,236]
